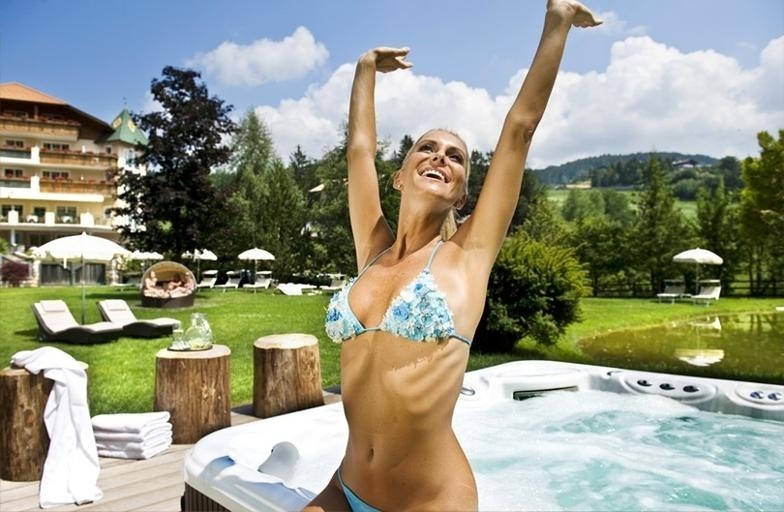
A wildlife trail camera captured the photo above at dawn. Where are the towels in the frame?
[9,347,173,509]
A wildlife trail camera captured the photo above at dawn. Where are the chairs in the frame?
[322,272,345,294]
[111,272,143,292]
[657,278,722,307]
[198,269,274,293]
[31,297,182,345]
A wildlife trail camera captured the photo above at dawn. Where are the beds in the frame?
[140,261,196,307]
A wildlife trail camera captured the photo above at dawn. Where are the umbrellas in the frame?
[31,231,132,325]
[127,247,164,271]
[672,246,723,296]
[237,247,275,281]
[672,325,725,370]
[180,247,217,282]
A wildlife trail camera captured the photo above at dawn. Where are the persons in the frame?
[164,278,190,297]
[184,271,194,291]
[299,0,606,512]
[144,271,159,297]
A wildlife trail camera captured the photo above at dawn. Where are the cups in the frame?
[172,321,184,344]
